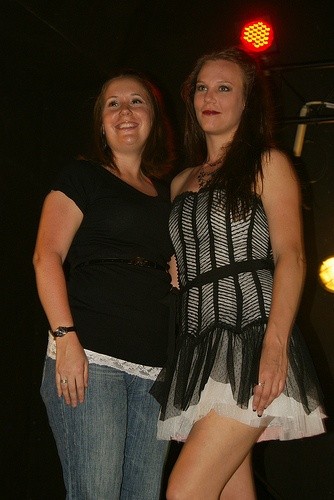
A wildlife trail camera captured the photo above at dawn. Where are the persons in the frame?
[151,49,326,500]
[32,67,191,500]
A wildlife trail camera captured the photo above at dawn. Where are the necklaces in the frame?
[196,152,229,187]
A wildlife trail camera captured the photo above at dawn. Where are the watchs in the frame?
[50,325,78,338]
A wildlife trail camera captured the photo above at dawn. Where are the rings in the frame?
[254,383,264,387]
[60,380,67,383]
